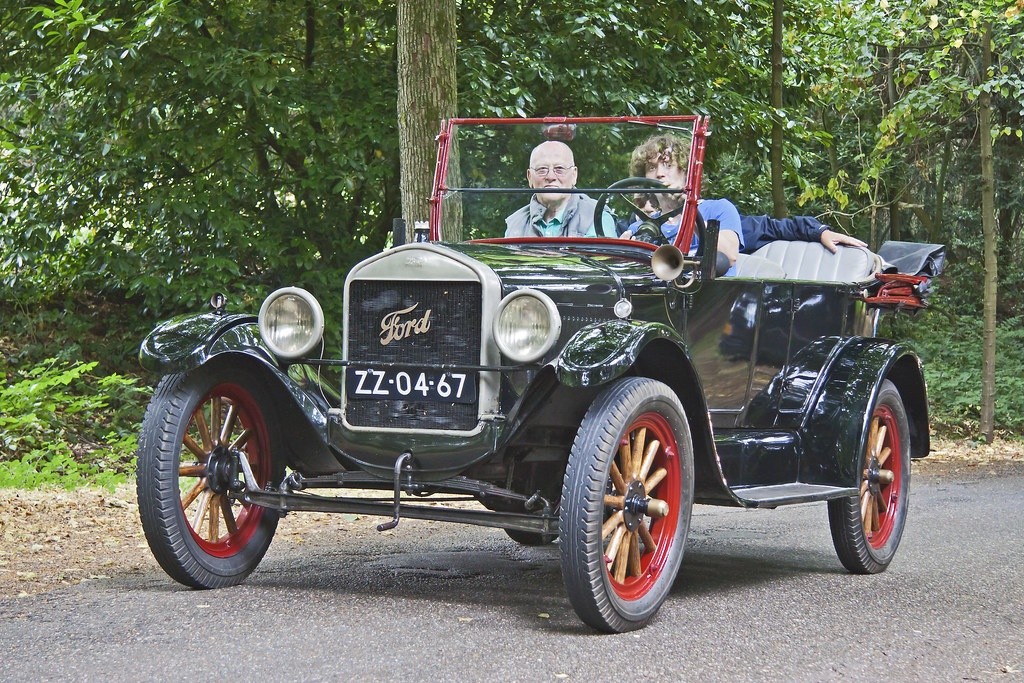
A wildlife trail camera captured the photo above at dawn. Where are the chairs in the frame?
[737,252,787,279]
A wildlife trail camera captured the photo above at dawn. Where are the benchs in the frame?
[748,240,876,284]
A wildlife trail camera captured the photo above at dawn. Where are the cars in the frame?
[135,116,948,634]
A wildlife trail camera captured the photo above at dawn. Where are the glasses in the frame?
[529,165,574,177]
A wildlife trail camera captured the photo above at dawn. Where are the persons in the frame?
[502,134,869,278]
